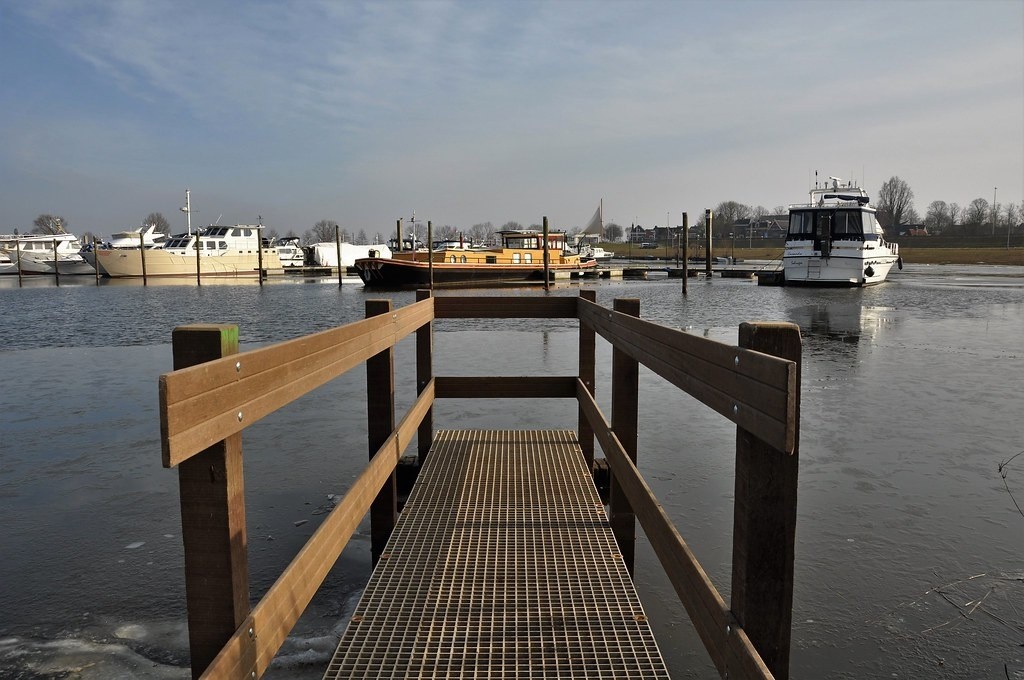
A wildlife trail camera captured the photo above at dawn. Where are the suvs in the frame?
[641,243,658,249]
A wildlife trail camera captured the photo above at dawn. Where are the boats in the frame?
[0,189,392,277]
[354,209,615,288]
[783,169,899,287]
